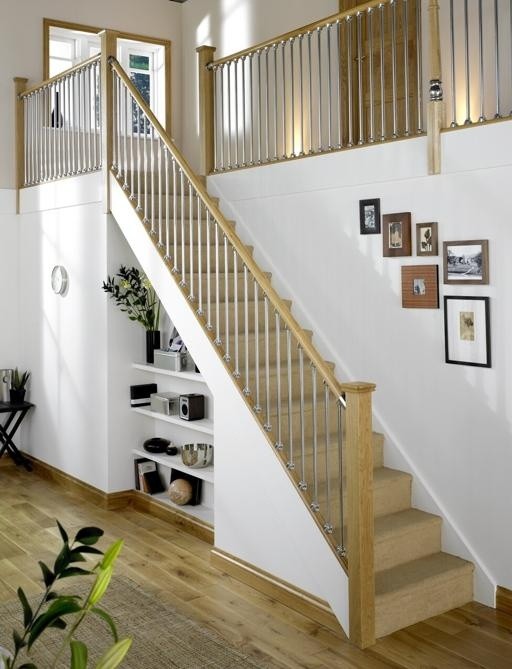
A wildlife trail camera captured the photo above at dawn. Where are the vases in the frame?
[146,330,160,363]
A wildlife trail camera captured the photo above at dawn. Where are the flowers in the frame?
[102,263,161,330]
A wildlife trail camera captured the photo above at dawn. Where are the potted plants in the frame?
[9,367,31,403]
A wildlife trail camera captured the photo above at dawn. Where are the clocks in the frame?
[51,265,67,295]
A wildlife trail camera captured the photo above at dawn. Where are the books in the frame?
[169,468,202,505]
[133,457,166,495]
[130,383,159,408]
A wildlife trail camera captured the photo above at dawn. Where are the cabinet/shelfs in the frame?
[131,363,214,526]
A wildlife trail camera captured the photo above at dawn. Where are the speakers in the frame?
[179,393,205,421]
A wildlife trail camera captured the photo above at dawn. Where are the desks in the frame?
[0,401,34,472]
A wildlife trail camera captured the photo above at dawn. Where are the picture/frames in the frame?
[360,198,491,368]
